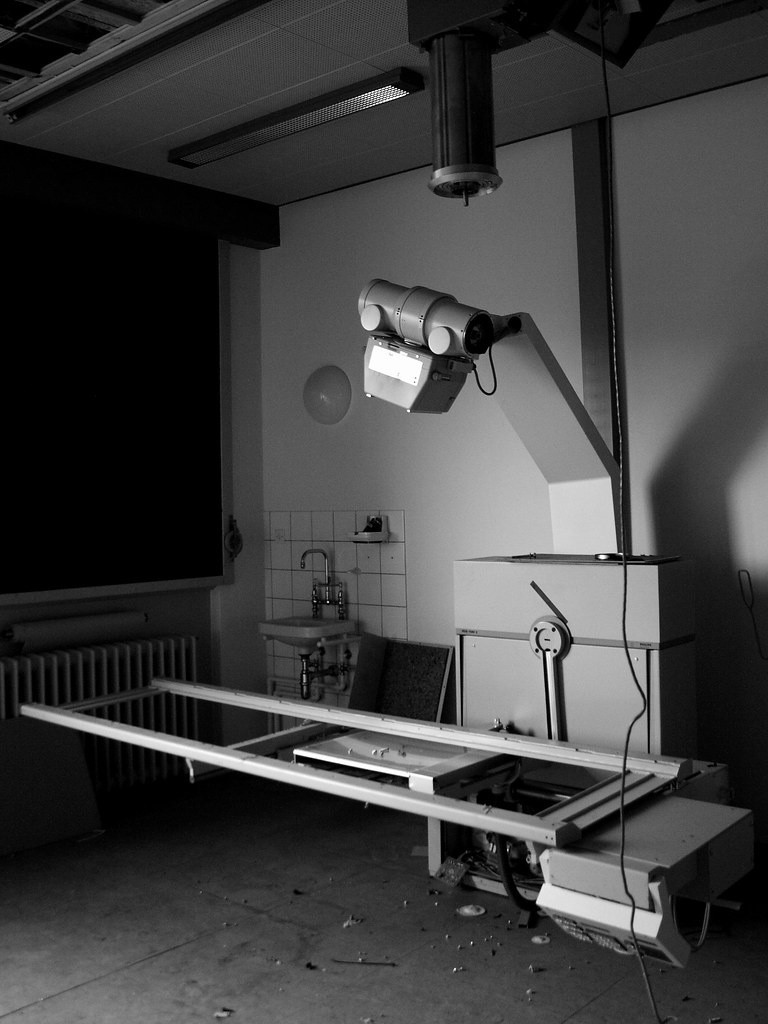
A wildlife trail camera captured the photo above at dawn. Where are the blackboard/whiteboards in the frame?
[0,223,235,608]
[346,632,454,722]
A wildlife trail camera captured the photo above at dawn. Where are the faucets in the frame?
[300,547,332,606]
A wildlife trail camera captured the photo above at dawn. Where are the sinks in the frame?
[259,615,357,648]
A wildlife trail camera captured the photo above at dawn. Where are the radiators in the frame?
[0,627,202,790]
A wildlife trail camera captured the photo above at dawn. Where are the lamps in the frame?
[166,66,427,168]
[302,364,351,427]
